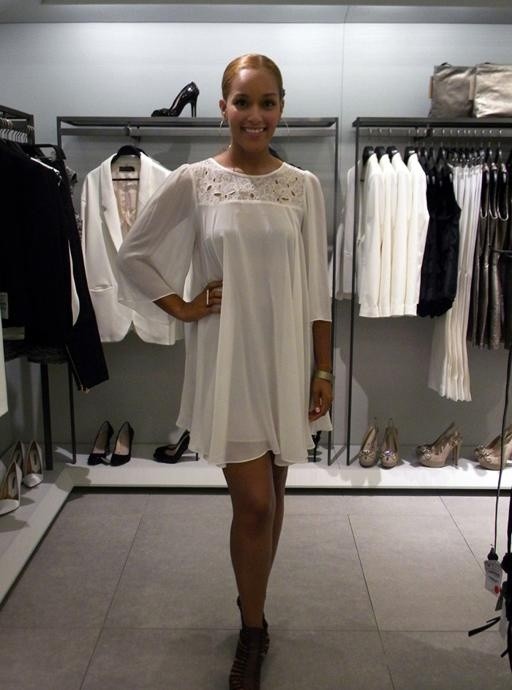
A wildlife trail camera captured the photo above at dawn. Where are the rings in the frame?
[205,288,212,307]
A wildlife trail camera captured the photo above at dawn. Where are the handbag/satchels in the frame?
[474,64,512,119]
[430,65,473,119]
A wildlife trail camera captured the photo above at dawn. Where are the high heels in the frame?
[88,420,113,465]
[111,421,134,465]
[416,421,463,467]
[312,432,322,461]
[153,430,198,463]
[151,82,199,117]
[475,425,512,470]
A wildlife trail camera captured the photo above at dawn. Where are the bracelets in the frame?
[311,368,335,385]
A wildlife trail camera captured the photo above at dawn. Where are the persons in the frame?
[115,53,335,689]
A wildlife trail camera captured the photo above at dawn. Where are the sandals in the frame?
[230,595,269,690]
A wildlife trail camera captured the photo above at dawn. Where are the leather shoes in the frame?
[360,426,399,467]
[0,439,44,515]
